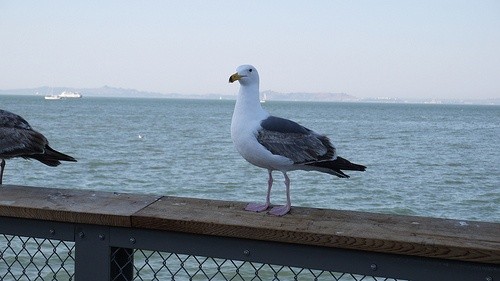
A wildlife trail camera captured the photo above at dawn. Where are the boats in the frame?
[58,90,81,97]
[45,95,61,99]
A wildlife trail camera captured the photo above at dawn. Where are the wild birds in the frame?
[229,65,367,216]
[0,108,78,186]
